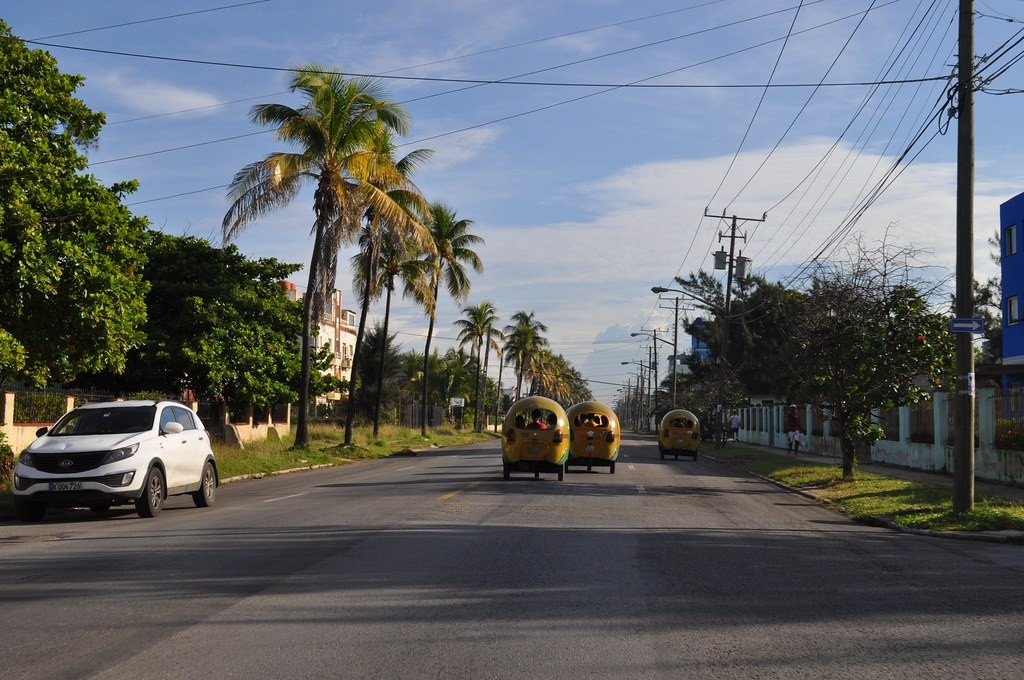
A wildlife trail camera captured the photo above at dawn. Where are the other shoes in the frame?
[736,437,740,441]
[732,440,736,442]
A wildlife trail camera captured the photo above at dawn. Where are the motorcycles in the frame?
[502,396,570,480]
[658,409,701,462]
[564,401,620,474]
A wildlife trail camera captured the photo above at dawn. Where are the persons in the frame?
[785,404,801,455]
[516,409,556,430]
[729,410,740,442]
[670,419,693,428]
[574,412,608,428]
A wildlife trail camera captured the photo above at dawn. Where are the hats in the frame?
[789,404,796,408]
[733,409,737,412]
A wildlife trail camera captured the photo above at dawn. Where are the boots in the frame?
[795,440,799,454]
[788,441,793,455]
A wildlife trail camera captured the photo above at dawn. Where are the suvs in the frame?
[9,400,220,518]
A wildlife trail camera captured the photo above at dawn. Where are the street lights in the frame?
[650,287,731,449]
[621,362,659,429]
[631,332,676,408]
